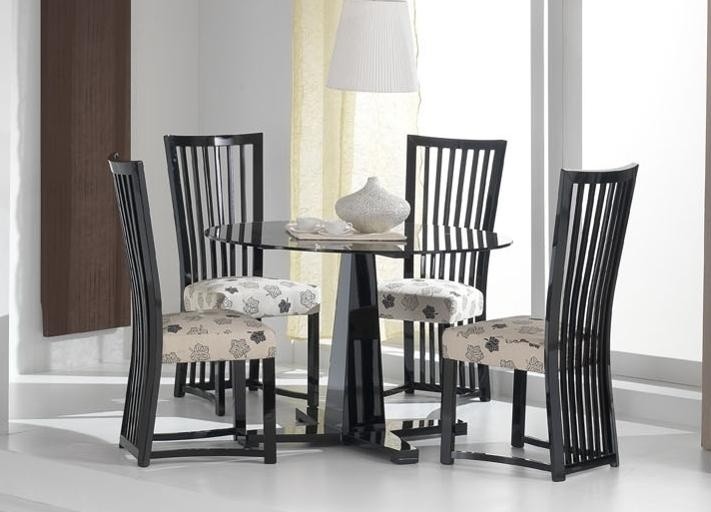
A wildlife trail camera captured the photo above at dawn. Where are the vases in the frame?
[335,176,412,234]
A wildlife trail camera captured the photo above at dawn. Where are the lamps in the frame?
[326,0,414,93]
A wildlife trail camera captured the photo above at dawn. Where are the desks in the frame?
[204,222,514,464]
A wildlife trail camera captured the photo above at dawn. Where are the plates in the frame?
[318,230,352,236]
[290,227,324,232]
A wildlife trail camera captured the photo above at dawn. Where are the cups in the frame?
[324,219,353,234]
[296,217,324,231]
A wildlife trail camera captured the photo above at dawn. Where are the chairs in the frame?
[378,135,506,402]
[441,163,639,482]
[164,132,322,416]
[107,152,278,467]
